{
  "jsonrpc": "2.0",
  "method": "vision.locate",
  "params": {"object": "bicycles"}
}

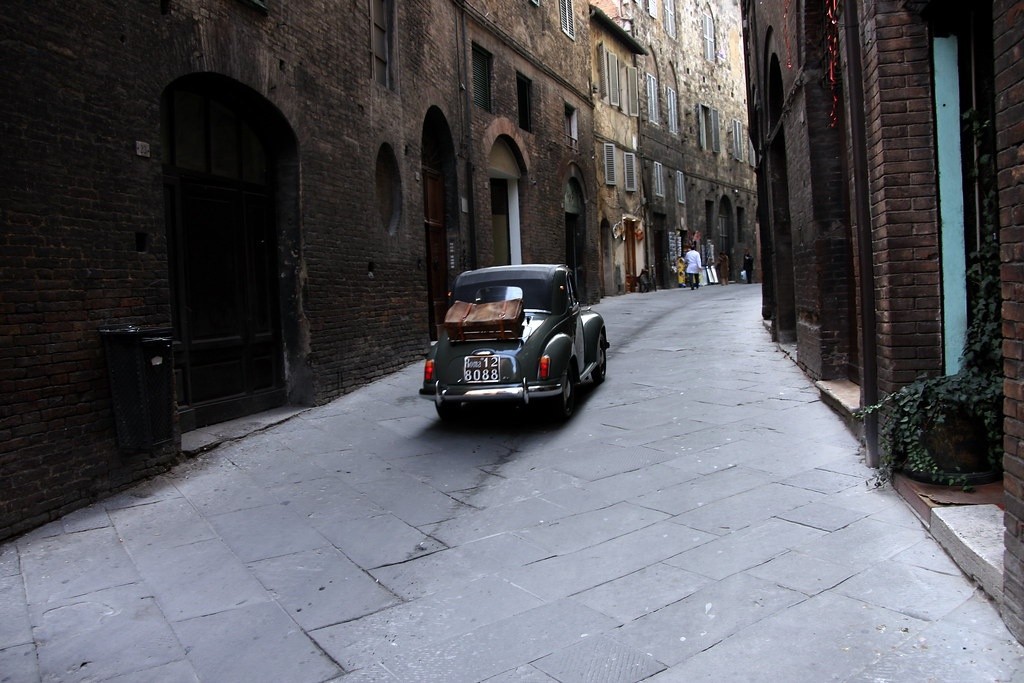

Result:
[636,264,656,292]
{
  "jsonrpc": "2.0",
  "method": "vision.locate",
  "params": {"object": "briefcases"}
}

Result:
[444,298,525,344]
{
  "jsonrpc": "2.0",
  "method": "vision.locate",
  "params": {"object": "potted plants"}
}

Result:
[852,87,1004,492]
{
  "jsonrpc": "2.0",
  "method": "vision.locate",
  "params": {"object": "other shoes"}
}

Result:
[692,285,694,290]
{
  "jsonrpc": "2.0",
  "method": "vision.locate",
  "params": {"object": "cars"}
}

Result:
[417,264,610,423]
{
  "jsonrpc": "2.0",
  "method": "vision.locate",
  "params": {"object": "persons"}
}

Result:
[683,245,702,290]
[716,252,730,285]
[742,248,755,284]
